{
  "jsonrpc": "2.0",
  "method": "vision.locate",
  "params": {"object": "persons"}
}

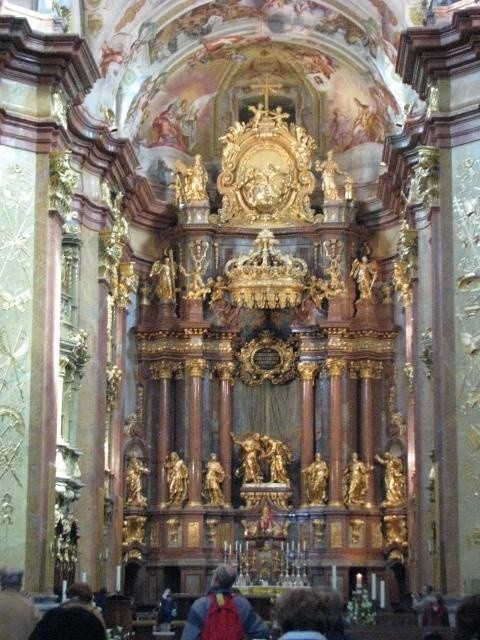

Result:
[316,152,344,201]
[123,436,409,556]
[353,257,374,298]
[181,267,224,299]
[0,564,480,640]
[181,153,209,201]
[155,258,172,300]
[306,269,343,305]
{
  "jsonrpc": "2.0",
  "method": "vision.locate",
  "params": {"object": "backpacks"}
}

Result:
[201,594,244,640]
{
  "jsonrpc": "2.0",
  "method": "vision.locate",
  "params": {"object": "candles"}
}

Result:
[368,573,377,598]
[331,564,338,592]
[354,570,365,593]
[113,565,123,593]
[59,578,66,599]
[379,579,384,609]
[219,537,312,558]
